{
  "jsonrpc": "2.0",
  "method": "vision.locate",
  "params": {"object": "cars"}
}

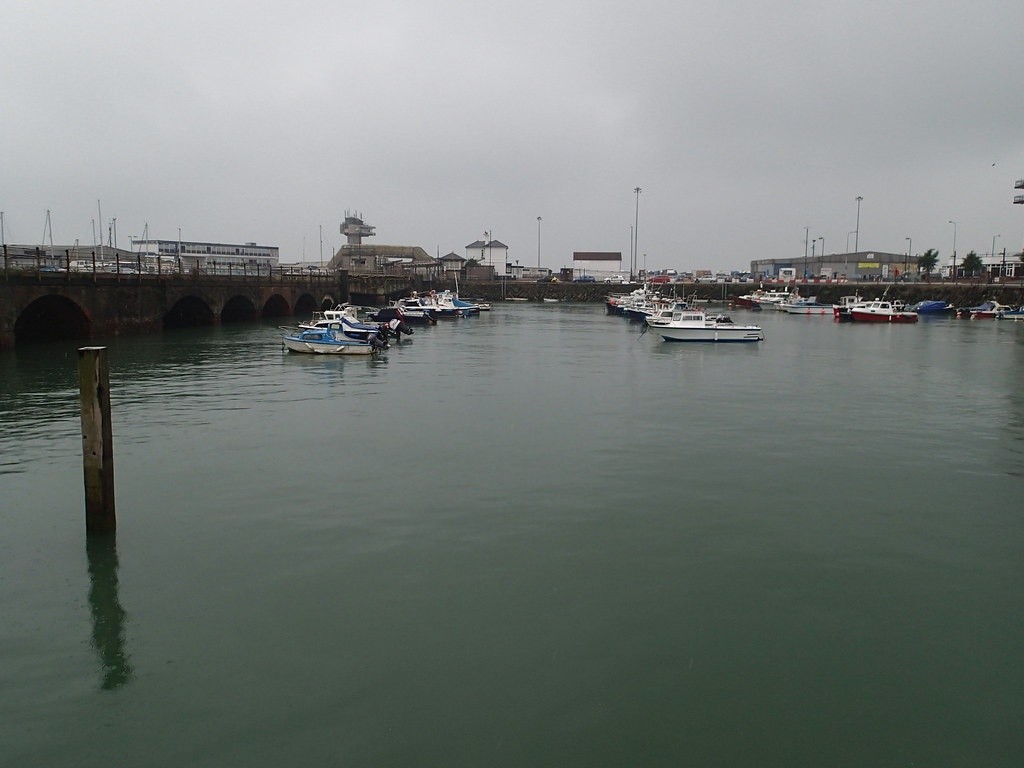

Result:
[693,275,717,284]
[604,275,624,284]
[537,276,557,283]
[572,275,595,283]
[647,275,670,284]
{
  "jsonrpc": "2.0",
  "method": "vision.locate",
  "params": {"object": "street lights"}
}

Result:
[948,220,956,251]
[992,234,1000,256]
[905,237,912,262]
[845,231,858,263]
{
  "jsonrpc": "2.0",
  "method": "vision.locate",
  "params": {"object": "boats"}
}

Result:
[298,310,382,340]
[783,296,833,314]
[650,310,762,341]
[970,305,1013,318]
[774,286,817,311]
[956,301,1000,315]
[622,296,674,320]
[760,291,790,303]
[733,289,766,305]
[616,293,639,315]
[903,301,954,313]
[645,302,716,327]
[284,323,375,354]
[604,292,629,314]
[424,289,480,317]
[833,295,863,316]
[996,306,1024,319]
[365,291,436,324]
[849,280,919,323]
